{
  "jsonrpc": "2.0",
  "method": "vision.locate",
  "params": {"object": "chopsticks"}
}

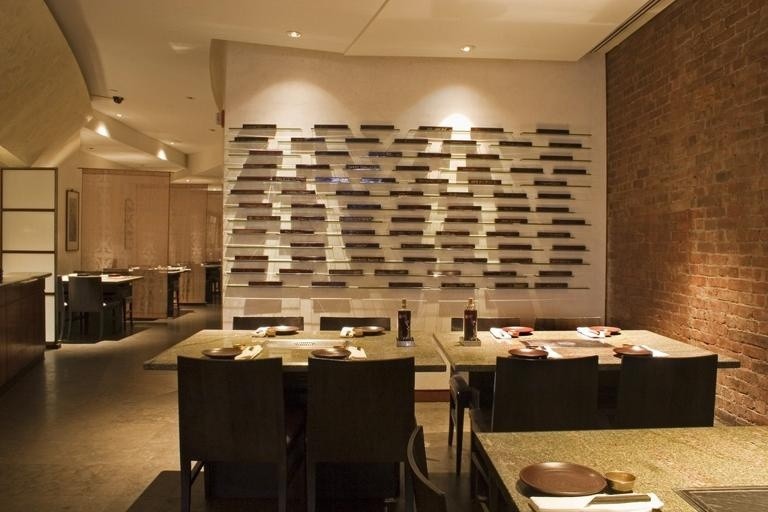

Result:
[591,494,652,503]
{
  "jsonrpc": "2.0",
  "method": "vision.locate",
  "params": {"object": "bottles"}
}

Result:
[397,300,414,341]
[463,298,480,339]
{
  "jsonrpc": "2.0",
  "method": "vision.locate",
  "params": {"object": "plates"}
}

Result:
[509,346,547,359]
[352,325,384,333]
[613,347,650,356]
[590,324,620,335]
[503,325,535,336]
[265,324,297,334]
[310,346,350,358]
[518,460,608,498]
[202,347,242,358]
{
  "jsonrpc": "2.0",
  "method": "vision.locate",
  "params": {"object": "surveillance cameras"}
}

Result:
[113,95,124,104]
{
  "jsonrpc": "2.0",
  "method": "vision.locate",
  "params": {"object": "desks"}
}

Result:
[434,330,741,412]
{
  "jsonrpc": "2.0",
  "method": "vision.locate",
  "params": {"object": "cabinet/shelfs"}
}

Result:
[0,275,48,388]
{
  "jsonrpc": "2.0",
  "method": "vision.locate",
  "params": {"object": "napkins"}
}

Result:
[577,327,604,338]
[530,493,664,511]
[489,327,511,339]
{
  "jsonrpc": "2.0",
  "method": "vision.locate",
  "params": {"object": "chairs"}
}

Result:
[449,319,522,484]
[611,355,717,429]
[55,261,221,344]
[470,355,602,509]
[178,315,449,511]
[534,319,601,331]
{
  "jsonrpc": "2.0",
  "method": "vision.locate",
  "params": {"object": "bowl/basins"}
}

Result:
[604,471,637,492]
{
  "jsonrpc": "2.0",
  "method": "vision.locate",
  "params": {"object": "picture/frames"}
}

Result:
[65,189,79,252]
